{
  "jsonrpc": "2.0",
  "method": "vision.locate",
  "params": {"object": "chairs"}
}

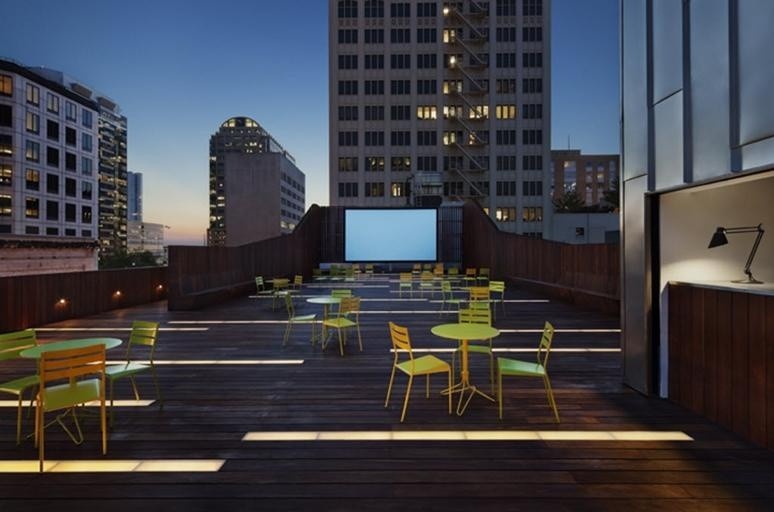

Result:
[0,319,160,474]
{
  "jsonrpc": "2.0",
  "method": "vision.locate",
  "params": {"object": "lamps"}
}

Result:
[706,223,765,285]
